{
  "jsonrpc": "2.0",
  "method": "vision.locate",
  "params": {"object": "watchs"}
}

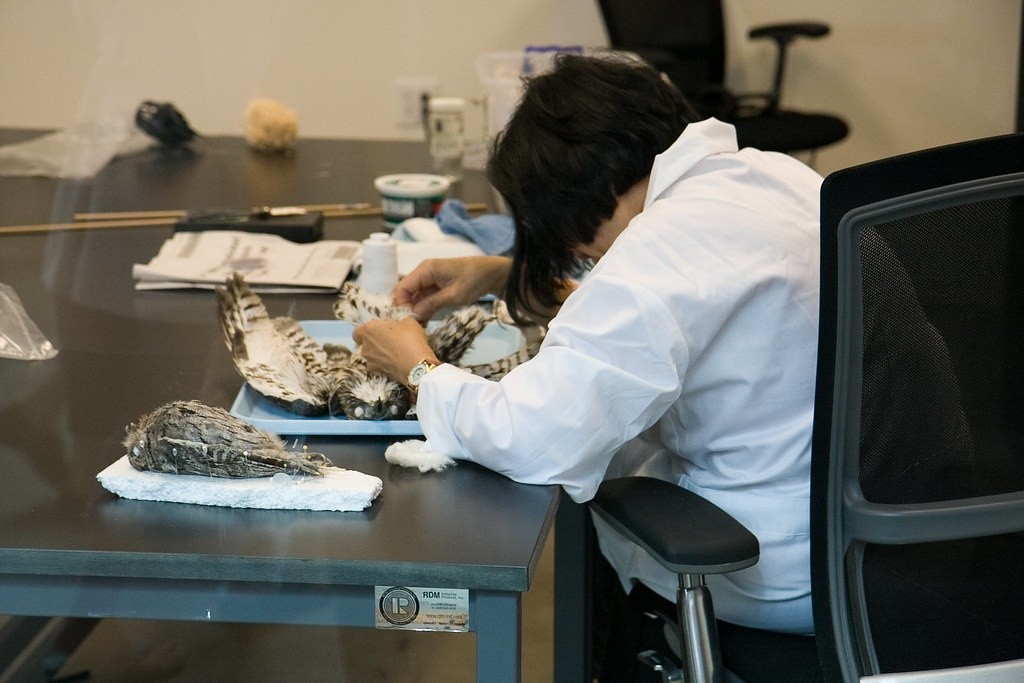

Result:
[407,358,442,402]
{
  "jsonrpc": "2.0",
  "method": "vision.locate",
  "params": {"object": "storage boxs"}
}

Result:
[475,49,646,141]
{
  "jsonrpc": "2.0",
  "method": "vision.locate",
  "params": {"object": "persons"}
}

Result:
[354,50,825,683]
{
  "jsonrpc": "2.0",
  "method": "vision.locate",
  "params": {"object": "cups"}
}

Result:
[354,232,400,322]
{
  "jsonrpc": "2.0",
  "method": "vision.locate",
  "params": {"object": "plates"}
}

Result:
[228,320,521,436]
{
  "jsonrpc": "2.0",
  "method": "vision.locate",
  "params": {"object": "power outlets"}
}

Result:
[390,82,435,126]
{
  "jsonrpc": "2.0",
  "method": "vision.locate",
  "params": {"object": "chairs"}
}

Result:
[592,134,1024,682]
[595,0,849,152]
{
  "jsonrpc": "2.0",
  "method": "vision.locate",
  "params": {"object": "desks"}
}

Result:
[0,126,604,683]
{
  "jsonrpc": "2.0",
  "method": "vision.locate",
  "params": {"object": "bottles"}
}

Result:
[427,97,466,158]
